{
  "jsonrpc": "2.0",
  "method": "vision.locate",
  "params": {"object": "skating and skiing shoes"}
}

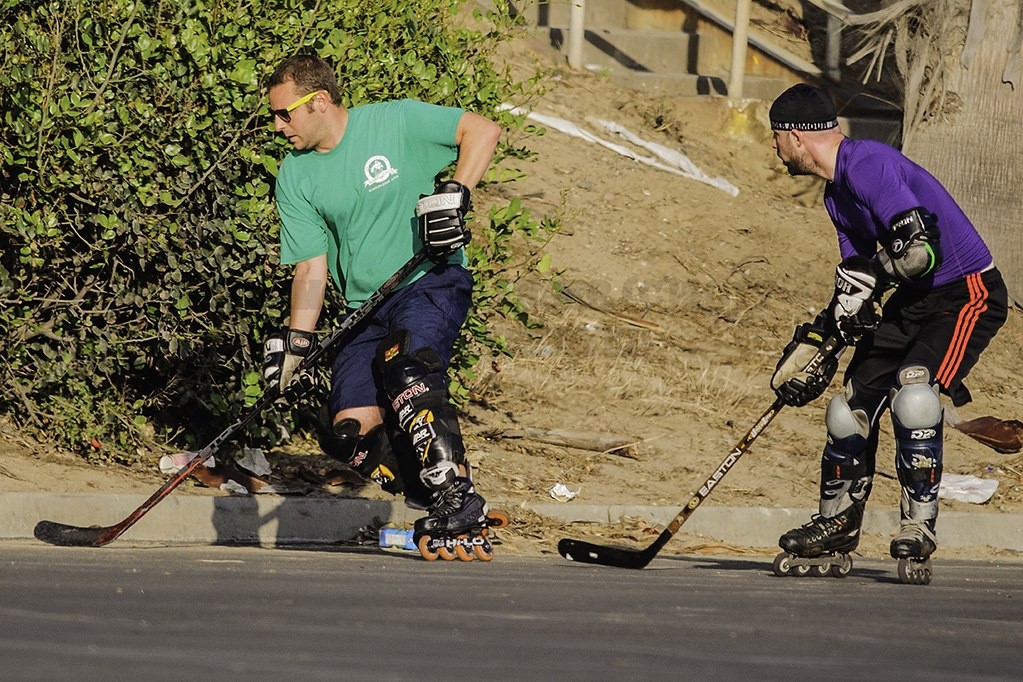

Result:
[476,494,511,531]
[773,513,860,579]
[413,491,490,559]
[890,512,939,585]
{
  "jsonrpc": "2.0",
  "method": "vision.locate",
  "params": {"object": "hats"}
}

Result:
[769,83,838,132]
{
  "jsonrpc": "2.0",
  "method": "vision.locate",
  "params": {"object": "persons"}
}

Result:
[262,53,509,564]
[763,84,1010,585]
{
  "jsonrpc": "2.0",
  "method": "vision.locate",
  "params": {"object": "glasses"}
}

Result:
[266,89,329,123]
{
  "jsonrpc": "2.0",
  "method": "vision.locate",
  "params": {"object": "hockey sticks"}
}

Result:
[554,332,841,571]
[32,246,428,549]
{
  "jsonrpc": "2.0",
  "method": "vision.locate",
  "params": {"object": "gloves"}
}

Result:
[262,326,319,413]
[770,308,848,407]
[414,179,472,266]
[832,255,884,347]
